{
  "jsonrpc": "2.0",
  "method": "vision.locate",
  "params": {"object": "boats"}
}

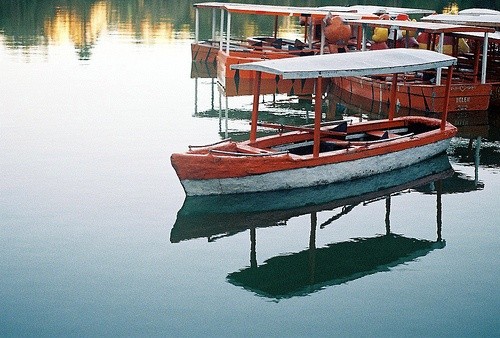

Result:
[217,75,329,141]
[326,82,488,195]
[191,1,500,112]
[191,60,218,119]
[170,48,458,197]
[169,149,456,299]
